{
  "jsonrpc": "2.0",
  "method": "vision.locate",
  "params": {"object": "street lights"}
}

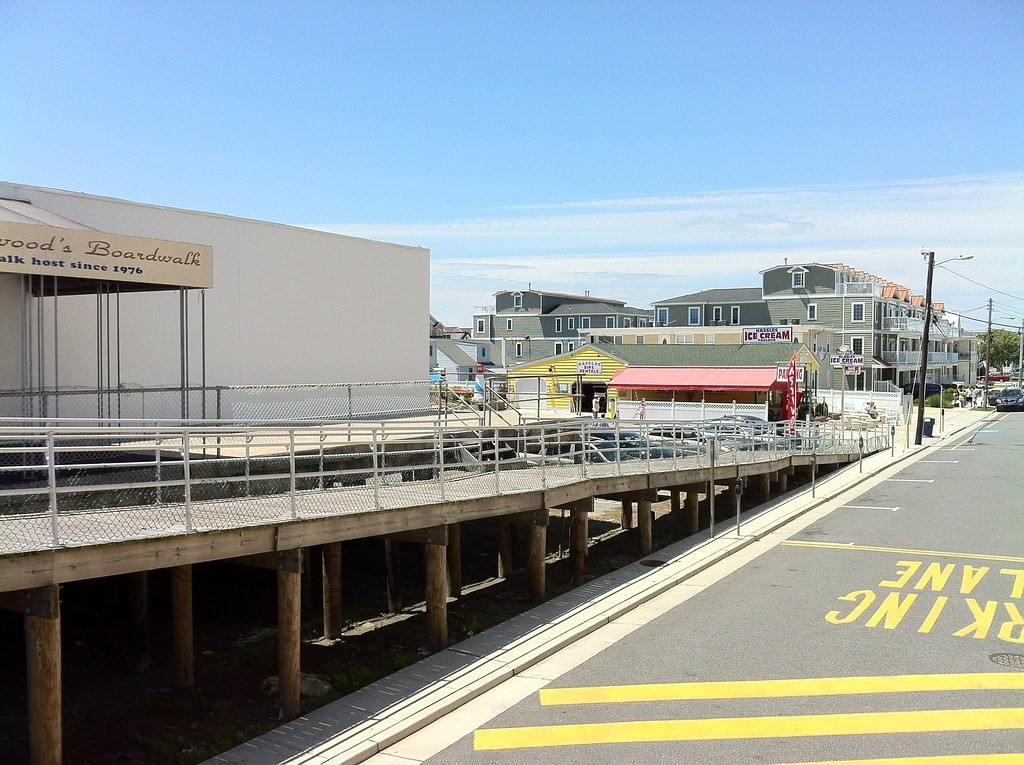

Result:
[983,316,1016,412]
[913,252,974,447]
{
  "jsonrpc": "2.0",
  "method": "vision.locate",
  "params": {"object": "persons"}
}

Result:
[613,410,619,419]
[637,400,645,420]
[592,395,601,419]
[866,401,878,419]
[953,383,986,409]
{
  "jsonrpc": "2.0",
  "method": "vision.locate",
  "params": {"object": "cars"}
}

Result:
[988,386,1024,412]
[977,372,1010,383]
[453,386,472,400]
[900,382,945,400]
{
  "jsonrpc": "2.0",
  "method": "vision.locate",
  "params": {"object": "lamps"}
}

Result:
[662,319,677,327]
[549,365,556,372]
[524,336,530,341]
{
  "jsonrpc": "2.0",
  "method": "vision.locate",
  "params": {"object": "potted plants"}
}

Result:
[462,397,473,409]
[466,387,474,398]
[447,392,455,402]
[450,385,462,391]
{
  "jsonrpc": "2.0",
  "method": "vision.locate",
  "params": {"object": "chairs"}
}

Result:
[827,413,887,432]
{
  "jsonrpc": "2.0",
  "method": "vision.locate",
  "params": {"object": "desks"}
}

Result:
[869,409,886,418]
[855,414,869,422]
[844,416,860,426]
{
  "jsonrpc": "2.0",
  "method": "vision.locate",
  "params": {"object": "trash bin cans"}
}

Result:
[923,417,935,437]
